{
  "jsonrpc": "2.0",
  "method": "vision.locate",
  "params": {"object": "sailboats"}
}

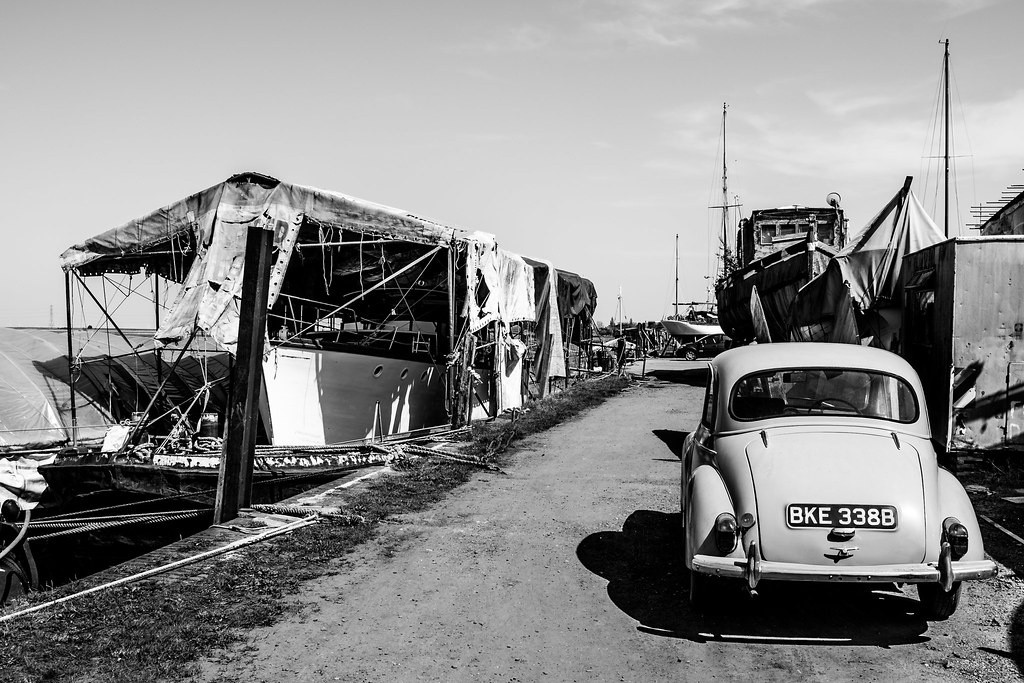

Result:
[662,102,729,341]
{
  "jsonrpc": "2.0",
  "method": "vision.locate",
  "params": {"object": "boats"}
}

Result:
[710,205,853,337]
[39,192,631,504]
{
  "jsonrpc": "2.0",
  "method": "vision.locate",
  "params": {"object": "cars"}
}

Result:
[681,343,997,619]
[675,334,736,360]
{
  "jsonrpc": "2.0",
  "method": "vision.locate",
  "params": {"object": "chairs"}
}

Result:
[732,397,784,419]
